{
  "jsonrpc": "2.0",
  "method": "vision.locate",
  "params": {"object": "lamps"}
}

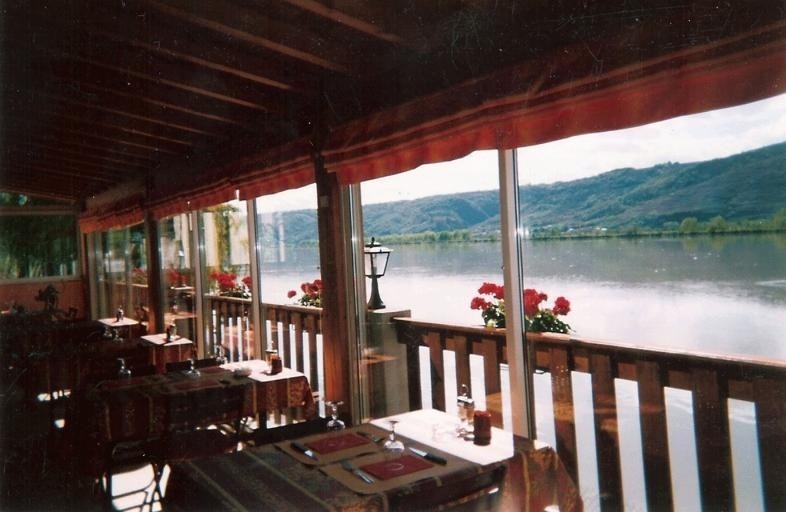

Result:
[363,237,394,310]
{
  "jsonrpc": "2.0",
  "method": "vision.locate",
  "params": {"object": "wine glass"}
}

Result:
[323,398,345,446]
[103,324,225,388]
[383,417,405,472]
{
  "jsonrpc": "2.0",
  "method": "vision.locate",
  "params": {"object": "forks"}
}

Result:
[356,429,385,443]
[342,459,375,485]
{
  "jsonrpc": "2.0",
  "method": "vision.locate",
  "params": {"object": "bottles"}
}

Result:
[453,385,492,446]
[115,305,126,322]
[263,341,282,375]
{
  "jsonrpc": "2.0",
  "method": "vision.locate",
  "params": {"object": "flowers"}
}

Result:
[287,279,323,308]
[471,282,577,336]
[208,269,253,299]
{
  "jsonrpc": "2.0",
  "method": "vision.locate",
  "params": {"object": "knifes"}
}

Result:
[408,444,448,466]
[289,439,319,462]
